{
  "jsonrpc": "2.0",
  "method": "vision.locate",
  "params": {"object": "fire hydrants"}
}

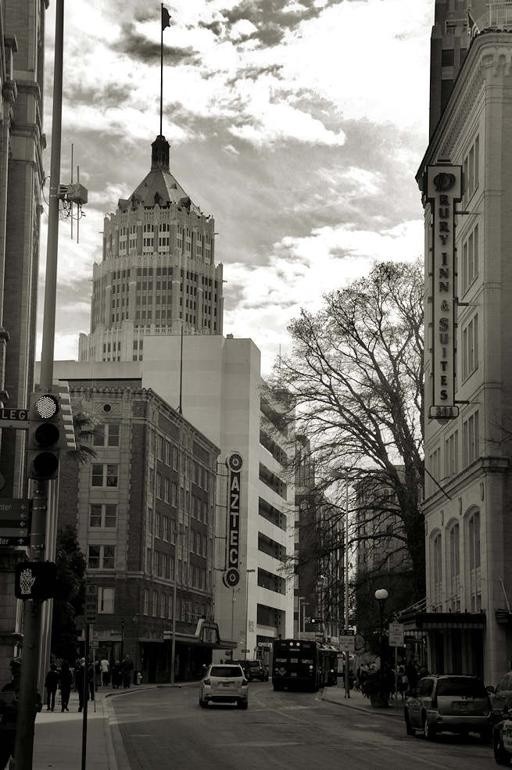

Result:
[137,672,143,684]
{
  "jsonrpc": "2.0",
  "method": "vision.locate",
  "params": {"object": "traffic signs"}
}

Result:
[0,497,29,545]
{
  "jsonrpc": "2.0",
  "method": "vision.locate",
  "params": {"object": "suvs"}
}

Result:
[199,658,269,709]
[402,672,494,741]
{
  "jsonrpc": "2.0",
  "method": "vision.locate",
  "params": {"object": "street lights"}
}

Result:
[373,585,395,710]
[328,469,351,692]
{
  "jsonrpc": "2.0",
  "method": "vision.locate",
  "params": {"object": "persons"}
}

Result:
[347,660,430,701]
[45,655,134,712]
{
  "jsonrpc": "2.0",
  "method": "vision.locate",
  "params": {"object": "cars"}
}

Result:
[487,670,512,767]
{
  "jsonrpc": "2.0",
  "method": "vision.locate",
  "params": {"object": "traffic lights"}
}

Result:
[13,559,61,601]
[25,391,63,482]
[310,616,321,624]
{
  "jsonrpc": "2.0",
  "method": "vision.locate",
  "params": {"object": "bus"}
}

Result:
[271,638,341,693]
